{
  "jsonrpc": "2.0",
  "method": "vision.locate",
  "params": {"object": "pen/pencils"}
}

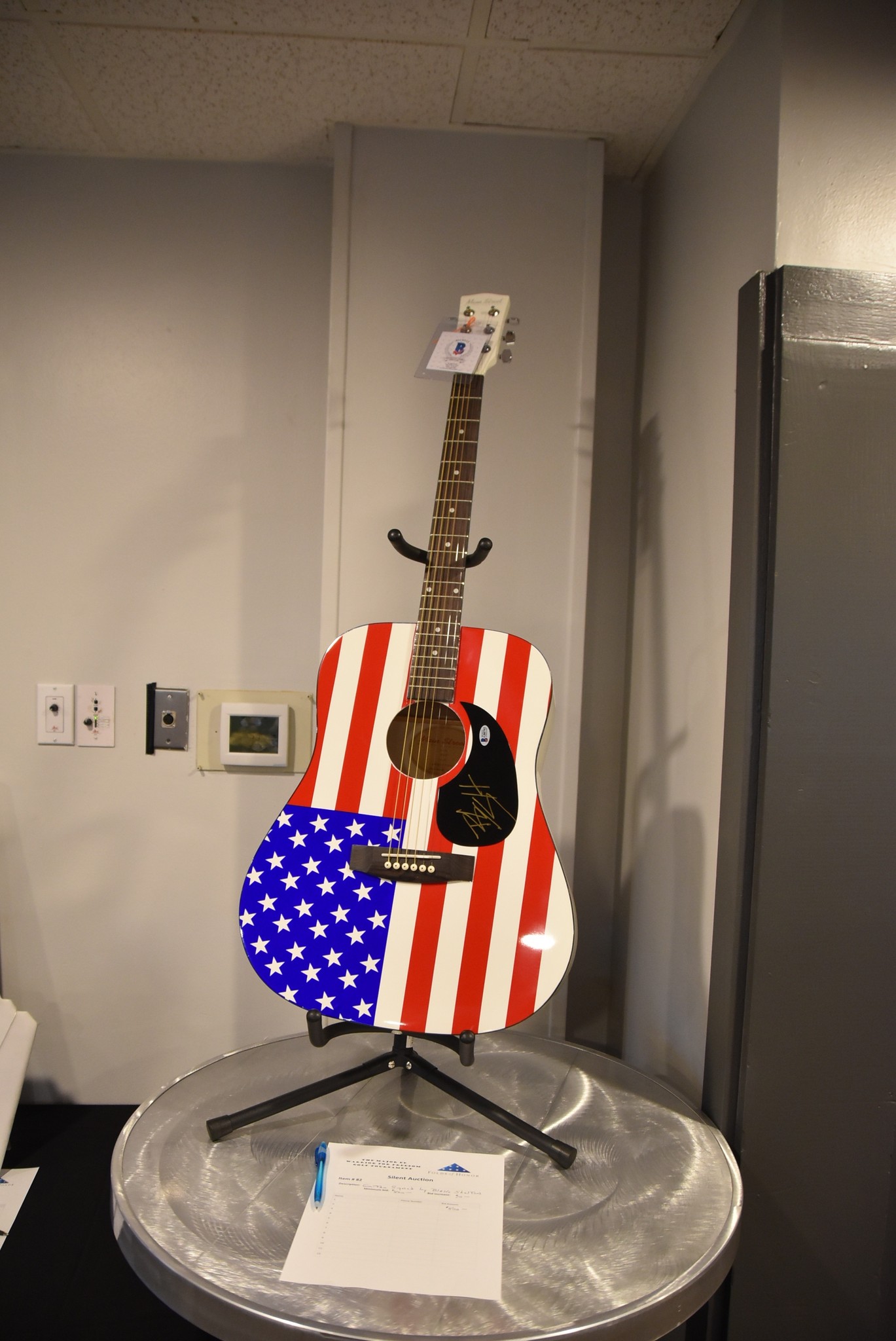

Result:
[314,1141,327,1210]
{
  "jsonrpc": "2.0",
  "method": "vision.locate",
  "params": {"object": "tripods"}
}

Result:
[205,526,580,1167]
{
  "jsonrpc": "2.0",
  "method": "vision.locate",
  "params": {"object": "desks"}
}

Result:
[113,1032,745,1341]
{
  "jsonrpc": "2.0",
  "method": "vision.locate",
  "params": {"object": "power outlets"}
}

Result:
[36,685,189,753]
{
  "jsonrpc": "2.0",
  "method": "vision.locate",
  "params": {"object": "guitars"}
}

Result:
[235,290,577,1046]
